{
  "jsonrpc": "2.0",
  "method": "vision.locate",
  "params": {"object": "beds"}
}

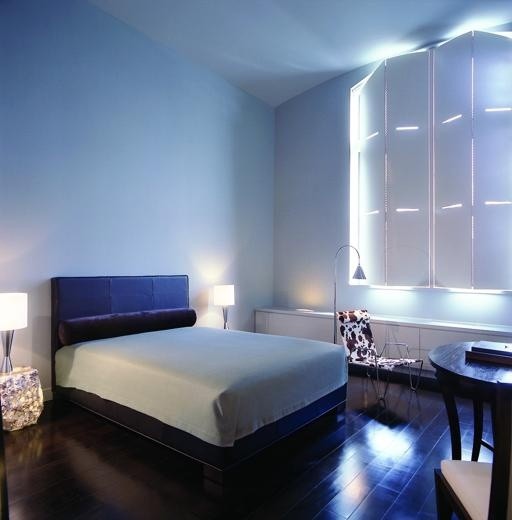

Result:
[51,275,347,484]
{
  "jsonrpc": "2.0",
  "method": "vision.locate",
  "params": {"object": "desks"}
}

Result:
[428,342,512,461]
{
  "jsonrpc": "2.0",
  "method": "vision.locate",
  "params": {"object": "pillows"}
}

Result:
[144,308,197,332]
[58,312,144,345]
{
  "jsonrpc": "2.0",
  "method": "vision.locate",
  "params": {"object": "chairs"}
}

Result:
[435,383,512,520]
[336,309,423,400]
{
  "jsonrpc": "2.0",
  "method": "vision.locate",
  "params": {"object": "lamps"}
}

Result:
[214,285,234,330]
[0,293,27,373]
[334,244,366,344]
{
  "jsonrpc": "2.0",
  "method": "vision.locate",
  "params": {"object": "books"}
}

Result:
[464,342,512,366]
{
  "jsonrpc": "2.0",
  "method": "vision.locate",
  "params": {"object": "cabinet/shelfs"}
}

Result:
[253,307,512,382]
